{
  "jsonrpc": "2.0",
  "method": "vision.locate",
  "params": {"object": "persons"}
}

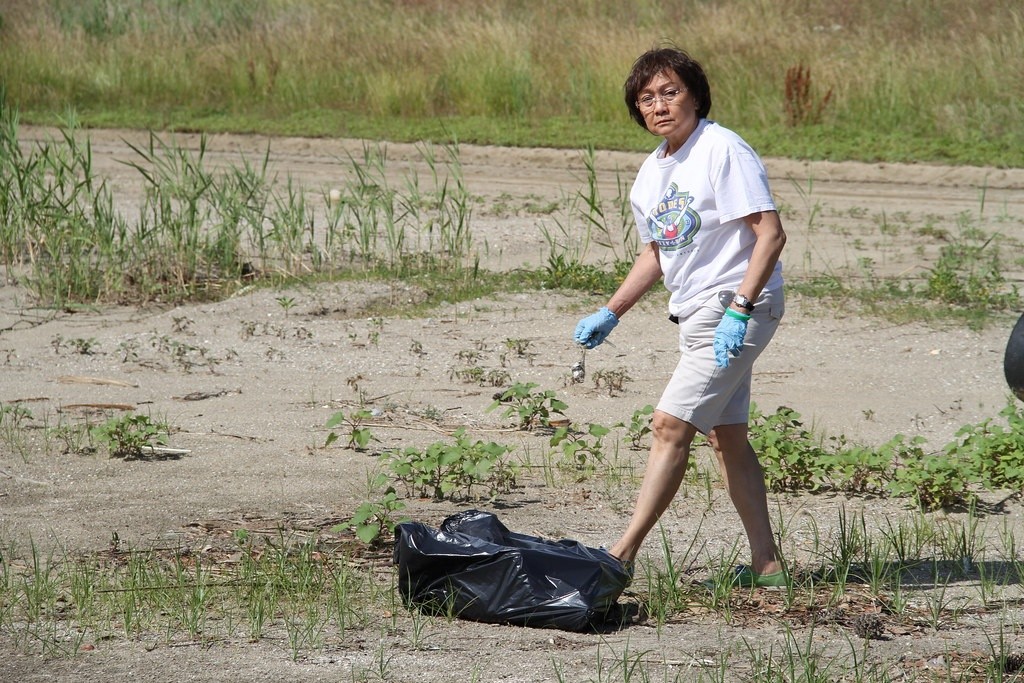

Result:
[575,48,789,589]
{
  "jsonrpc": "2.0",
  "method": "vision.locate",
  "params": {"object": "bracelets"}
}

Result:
[726,307,751,321]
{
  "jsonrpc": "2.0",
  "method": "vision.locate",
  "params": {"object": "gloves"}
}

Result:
[712,306,751,368]
[573,306,620,349]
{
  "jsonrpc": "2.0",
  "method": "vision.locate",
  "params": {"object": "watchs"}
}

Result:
[733,295,755,312]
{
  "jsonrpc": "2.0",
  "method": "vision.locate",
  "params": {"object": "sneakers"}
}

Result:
[621,561,635,587]
[704,565,792,591]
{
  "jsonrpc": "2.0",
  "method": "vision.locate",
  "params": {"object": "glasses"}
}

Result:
[634,87,689,109]
